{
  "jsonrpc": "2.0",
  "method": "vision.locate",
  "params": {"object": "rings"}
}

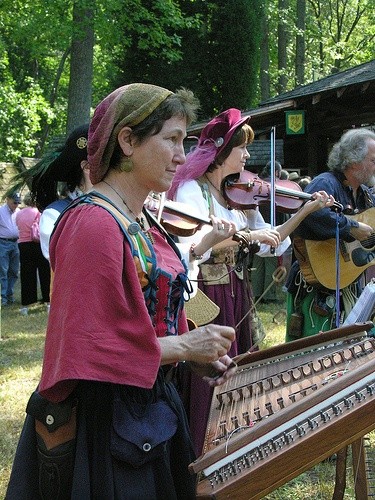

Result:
[218,226,223,230]
[225,227,230,230]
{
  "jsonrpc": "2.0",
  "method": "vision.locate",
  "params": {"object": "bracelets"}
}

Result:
[190,243,203,260]
[244,228,253,243]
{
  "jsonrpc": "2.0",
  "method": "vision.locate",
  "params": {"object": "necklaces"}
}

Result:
[101,179,155,245]
[204,172,233,210]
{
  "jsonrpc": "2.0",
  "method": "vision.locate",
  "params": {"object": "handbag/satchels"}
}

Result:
[25,390,80,500]
[30,212,41,242]
[109,382,179,468]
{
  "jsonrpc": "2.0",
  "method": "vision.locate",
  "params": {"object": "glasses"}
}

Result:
[14,202,21,204]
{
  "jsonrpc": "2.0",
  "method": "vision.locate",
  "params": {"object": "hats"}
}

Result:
[87,83,174,184]
[8,192,21,201]
[198,108,251,160]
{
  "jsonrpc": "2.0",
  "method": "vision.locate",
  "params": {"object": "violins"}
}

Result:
[225,168,354,218]
[143,195,261,256]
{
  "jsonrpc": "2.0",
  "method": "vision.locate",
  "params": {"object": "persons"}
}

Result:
[31,124,236,270]
[166,109,334,459]
[283,128,375,459]
[251,160,286,304]
[277,169,311,288]
[5,83,238,500]
[0,192,21,306]
[16,190,50,315]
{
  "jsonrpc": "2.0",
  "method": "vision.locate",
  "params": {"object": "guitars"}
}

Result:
[291,207,375,290]
